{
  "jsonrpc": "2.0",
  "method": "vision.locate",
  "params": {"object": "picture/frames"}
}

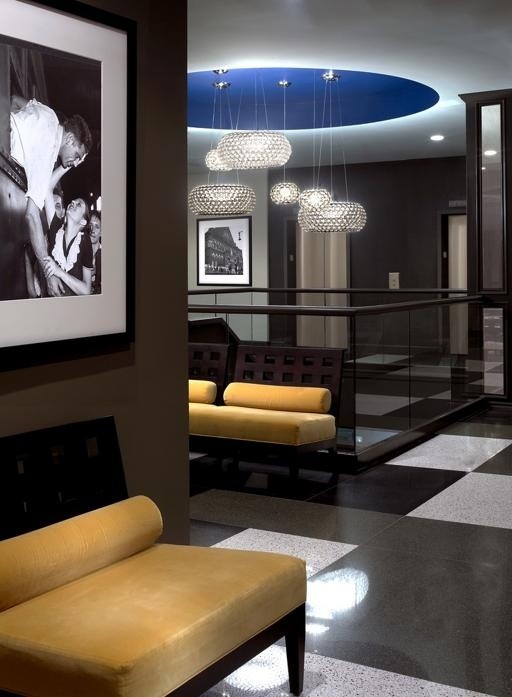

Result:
[0,0,140,373]
[195,214,253,288]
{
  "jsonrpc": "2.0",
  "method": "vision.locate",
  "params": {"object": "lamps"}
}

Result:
[188,65,368,233]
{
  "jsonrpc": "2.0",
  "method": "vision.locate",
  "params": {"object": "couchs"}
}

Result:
[1,414,307,695]
[188,340,345,488]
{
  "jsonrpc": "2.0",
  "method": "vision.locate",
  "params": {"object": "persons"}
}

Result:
[38,149,94,296]
[86,208,102,294]
[52,184,66,222]
[8,94,94,299]
